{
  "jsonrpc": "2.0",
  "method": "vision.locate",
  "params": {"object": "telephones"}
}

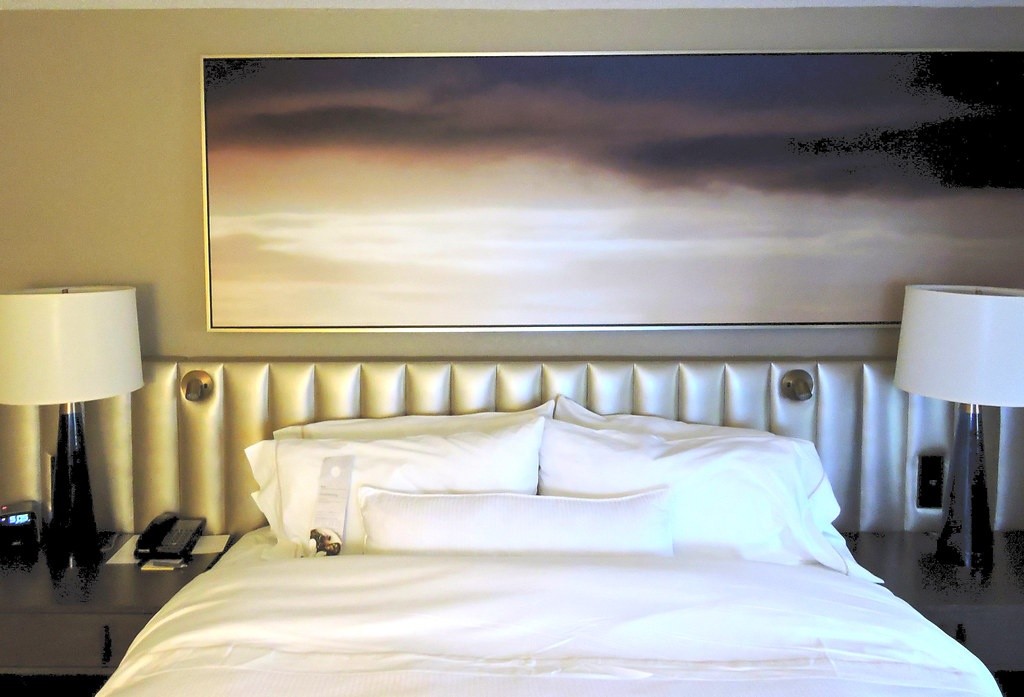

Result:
[133,510,207,560]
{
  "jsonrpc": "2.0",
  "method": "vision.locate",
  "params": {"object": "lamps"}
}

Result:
[891,283,1024,568]
[0,282,146,565]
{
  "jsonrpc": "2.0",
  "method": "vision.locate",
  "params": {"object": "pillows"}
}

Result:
[244,393,888,585]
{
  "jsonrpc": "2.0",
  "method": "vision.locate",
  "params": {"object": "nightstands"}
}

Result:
[0,532,237,697]
[840,530,1024,697]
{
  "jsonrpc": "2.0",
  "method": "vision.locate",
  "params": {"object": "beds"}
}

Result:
[94,522,1006,697]
